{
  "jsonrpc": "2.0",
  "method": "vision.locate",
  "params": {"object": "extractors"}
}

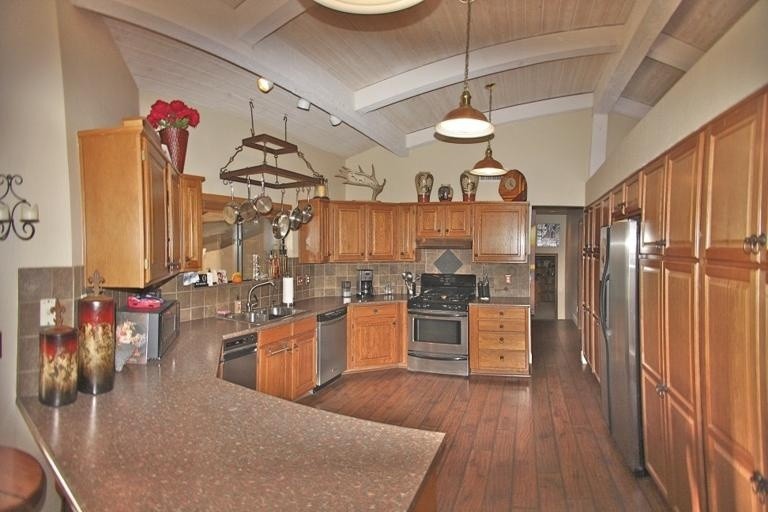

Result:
[416,237,472,249]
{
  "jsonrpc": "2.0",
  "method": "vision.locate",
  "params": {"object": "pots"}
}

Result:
[222,173,314,241]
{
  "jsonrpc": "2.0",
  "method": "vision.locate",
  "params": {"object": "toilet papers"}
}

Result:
[283,277,293,304]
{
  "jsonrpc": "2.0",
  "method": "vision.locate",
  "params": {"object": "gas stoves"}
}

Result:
[407,290,473,310]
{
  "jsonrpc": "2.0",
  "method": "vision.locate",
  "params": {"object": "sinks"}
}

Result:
[213,312,283,325]
[252,305,311,317]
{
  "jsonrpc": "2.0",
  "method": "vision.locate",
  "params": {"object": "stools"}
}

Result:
[0,445,46,512]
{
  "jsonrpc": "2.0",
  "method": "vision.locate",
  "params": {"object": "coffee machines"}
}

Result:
[355,269,374,296]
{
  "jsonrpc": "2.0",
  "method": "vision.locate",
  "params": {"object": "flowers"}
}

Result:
[147,100,200,129]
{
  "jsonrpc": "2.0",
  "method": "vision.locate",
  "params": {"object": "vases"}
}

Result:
[158,127,189,174]
[460,170,479,202]
[438,184,453,202]
[415,172,434,203]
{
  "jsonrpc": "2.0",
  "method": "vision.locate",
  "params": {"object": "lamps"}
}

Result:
[468,82,509,178]
[0,174,39,241]
[329,114,342,126]
[296,99,311,111]
[434,1,494,141]
[257,77,273,93]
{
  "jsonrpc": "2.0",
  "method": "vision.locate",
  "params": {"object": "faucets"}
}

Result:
[246,281,276,310]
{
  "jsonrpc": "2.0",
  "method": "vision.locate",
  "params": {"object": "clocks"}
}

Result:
[499,170,527,202]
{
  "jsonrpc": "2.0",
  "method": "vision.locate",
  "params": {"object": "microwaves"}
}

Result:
[115,299,181,360]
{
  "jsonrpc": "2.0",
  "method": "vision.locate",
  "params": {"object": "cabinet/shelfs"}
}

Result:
[254,316,317,401]
[472,201,530,262]
[416,202,472,240]
[346,301,407,374]
[78,116,167,288]
[167,160,181,277]
[330,201,365,264]
[469,303,531,378]
[365,202,396,263]
[298,200,331,264]
[395,203,421,263]
[181,173,207,273]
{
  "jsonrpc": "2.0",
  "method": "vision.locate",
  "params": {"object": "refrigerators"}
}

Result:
[599,218,645,477]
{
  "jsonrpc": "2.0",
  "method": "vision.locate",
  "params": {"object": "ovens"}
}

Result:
[407,308,469,377]
